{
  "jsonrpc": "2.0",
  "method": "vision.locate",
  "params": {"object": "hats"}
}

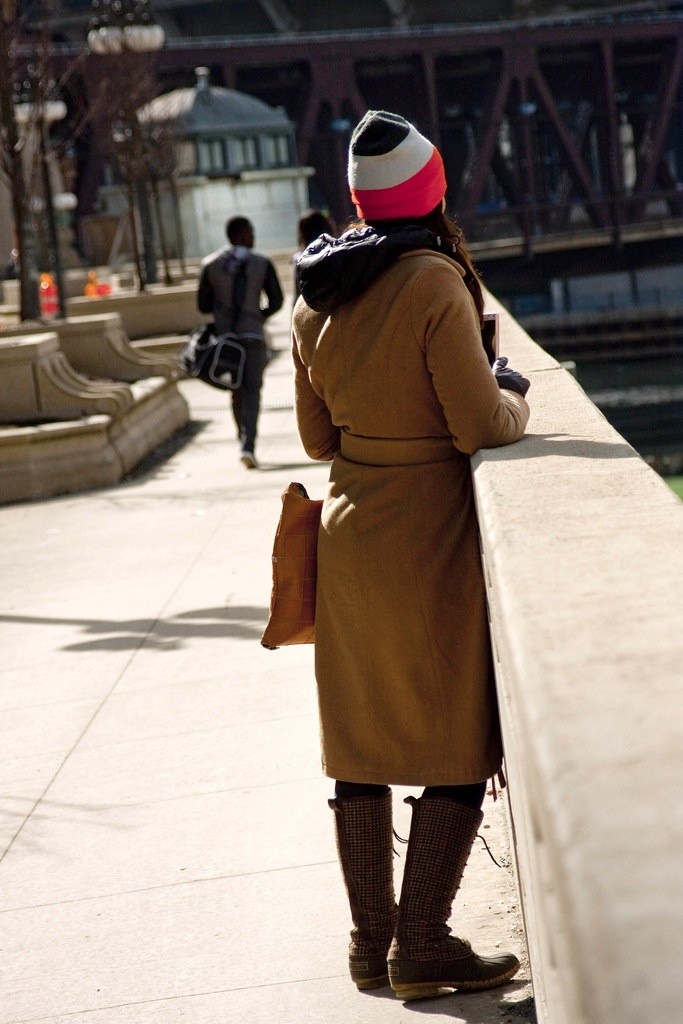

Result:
[348,110,447,220]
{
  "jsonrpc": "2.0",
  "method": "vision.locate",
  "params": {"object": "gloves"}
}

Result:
[491,357,531,399]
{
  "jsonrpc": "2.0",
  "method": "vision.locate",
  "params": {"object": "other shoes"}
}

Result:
[239,445,258,467]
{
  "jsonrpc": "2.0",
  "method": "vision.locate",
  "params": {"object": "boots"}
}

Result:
[327,790,400,990]
[385,795,519,998]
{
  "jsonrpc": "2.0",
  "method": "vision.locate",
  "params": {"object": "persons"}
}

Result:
[194,215,286,469]
[293,213,334,262]
[293,105,534,1007]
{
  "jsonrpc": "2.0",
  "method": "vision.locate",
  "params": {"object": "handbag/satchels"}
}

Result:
[260,482,324,650]
[178,334,245,390]
